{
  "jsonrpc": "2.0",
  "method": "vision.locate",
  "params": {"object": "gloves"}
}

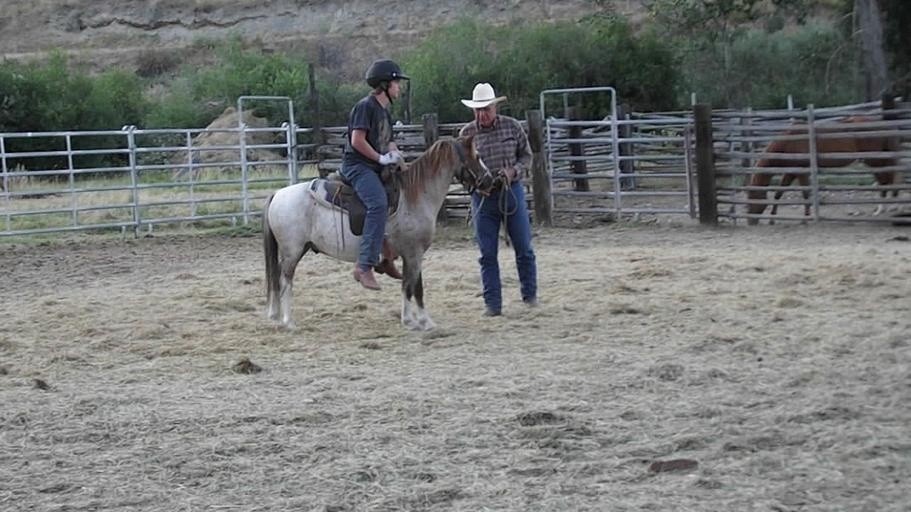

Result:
[378,150,405,167]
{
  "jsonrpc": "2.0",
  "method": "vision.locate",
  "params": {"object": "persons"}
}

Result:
[455,81,543,319]
[337,59,413,292]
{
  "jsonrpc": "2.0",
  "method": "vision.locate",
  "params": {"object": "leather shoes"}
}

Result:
[525,299,538,308]
[377,260,405,282]
[482,309,501,317]
[351,262,383,294]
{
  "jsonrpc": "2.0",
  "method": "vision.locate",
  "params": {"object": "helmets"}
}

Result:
[363,59,412,83]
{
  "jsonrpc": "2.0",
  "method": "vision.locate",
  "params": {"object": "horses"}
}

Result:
[263,134,495,331]
[747,116,899,224]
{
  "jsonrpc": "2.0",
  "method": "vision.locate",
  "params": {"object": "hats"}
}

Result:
[460,82,507,111]
